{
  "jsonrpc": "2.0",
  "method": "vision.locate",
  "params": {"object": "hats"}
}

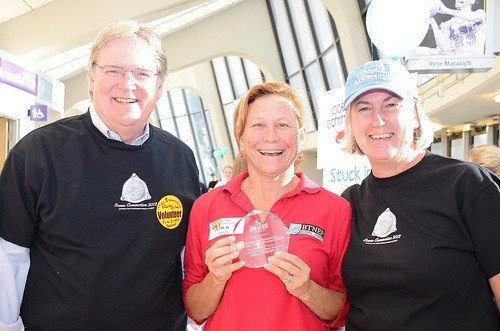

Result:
[343,59,420,110]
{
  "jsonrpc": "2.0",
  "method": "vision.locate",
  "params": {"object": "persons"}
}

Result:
[466,145,500,178]
[176,83,352,330]
[429,0,487,59]
[1,22,218,330]
[331,60,500,330]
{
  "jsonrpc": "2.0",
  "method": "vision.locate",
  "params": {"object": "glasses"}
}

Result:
[93,62,160,82]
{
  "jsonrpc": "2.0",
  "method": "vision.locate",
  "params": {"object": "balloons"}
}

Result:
[364,0,430,62]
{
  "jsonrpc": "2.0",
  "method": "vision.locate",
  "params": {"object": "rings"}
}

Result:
[283,276,293,282]
